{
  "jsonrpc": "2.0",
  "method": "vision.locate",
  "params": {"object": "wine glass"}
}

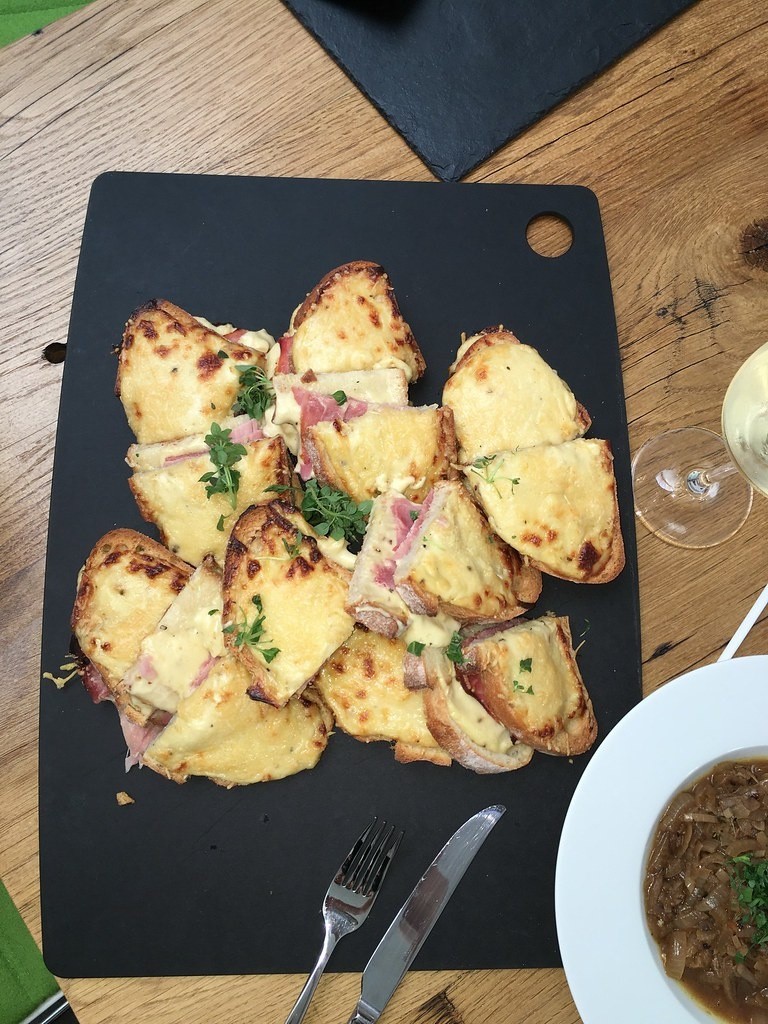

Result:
[627,337,768,550]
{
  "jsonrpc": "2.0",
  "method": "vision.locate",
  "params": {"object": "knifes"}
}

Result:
[339,803,508,1024]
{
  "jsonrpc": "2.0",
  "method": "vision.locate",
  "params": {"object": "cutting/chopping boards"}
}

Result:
[35,174,641,978]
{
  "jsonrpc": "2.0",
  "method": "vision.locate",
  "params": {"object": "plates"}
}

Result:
[554,654,768,1023]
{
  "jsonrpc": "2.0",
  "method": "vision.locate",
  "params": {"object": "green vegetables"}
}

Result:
[198,365,531,690]
[727,854,768,963]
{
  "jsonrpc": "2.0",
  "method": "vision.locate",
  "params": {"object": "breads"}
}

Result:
[318,620,452,768]
[423,634,532,771]
[463,441,625,583]
[305,408,456,510]
[457,617,598,756]
[396,480,543,619]
[66,528,196,720]
[116,298,268,443]
[291,262,426,382]
[140,657,331,788]
[128,429,305,565]
[443,331,592,454]
[220,500,360,710]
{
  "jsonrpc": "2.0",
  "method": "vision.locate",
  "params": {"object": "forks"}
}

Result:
[278,816,406,1024]
